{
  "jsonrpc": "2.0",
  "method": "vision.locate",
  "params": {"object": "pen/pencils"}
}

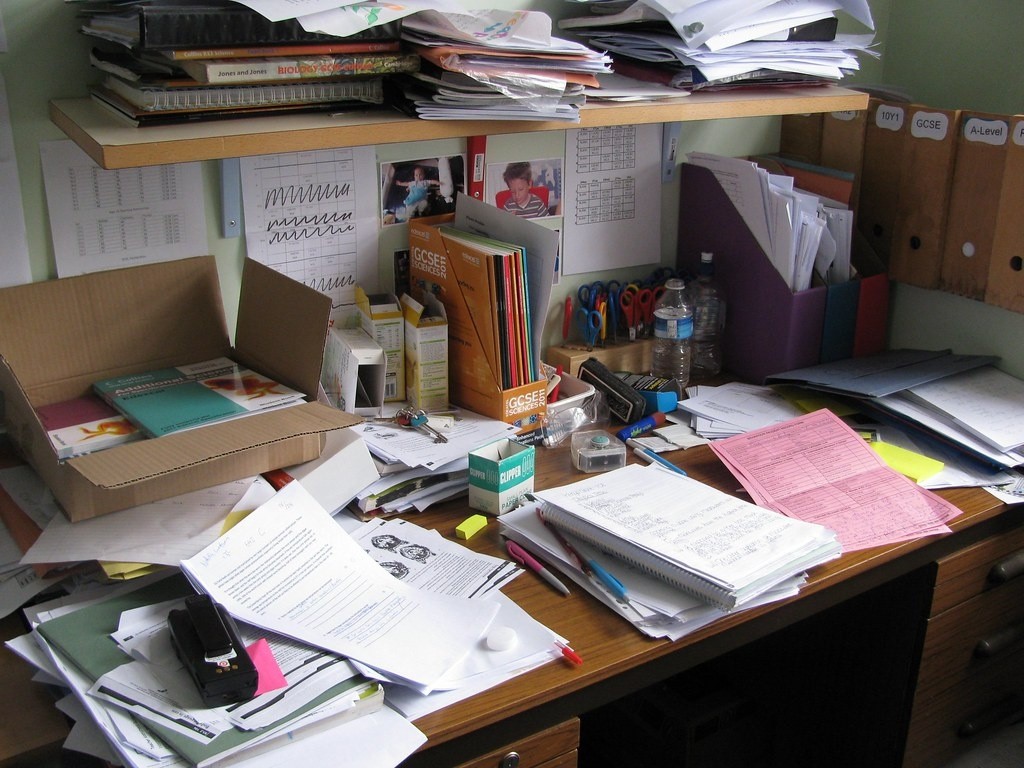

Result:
[554,637,582,665]
[535,506,631,603]
[626,437,687,476]
[505,540,573,598]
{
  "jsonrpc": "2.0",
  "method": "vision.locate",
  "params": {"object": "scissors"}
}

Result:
[560,267,695,350]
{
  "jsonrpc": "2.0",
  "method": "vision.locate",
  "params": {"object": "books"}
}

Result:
[23,568,384,767]
[35,356,310,461]
[350,454,471,514]
[83,0,421,129]
[540,462,843,612]
[434,223,534,391]
[863,391,1024,472]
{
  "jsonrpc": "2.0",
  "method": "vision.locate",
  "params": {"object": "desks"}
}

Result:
[0,445,1023,768]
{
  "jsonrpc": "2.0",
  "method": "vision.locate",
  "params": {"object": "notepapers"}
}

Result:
[867,441,945,484]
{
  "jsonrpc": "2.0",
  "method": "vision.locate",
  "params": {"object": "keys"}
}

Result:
[398,413,448,444]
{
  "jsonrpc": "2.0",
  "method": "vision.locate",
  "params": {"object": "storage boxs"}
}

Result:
[468,439,535,514]
[326,326,386,419]
[408,212,549,451]
[401,291,449,414]
[679,154,890,384]
[0,254,365,521]
[352,284,407,403]
[616,371,681,415]
[545,363,594,416]
[779,103,1023,314]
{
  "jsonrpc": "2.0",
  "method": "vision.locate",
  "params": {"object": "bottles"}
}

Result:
[650,278,694,389]
[688,251,726,379]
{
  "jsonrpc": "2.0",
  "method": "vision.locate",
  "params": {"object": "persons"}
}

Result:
[503,162,551,218]
[395,166,445,219]
